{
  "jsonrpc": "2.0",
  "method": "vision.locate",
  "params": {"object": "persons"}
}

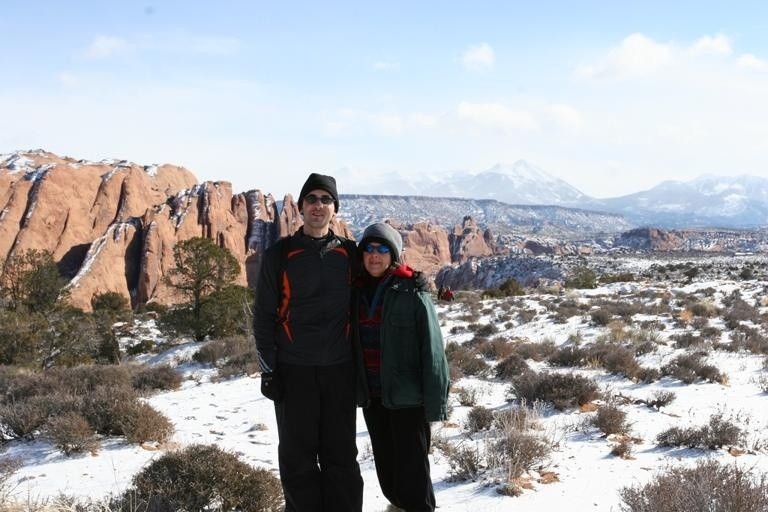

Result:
[251,171,434,510]
[438,283,445,300]
[348,220,451,510]
[441,285,454,301]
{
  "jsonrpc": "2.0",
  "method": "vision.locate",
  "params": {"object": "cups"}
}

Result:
[305,194,333,204]
[363,244,391,254]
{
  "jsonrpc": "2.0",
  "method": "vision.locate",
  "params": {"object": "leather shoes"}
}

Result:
[413,271,431,291]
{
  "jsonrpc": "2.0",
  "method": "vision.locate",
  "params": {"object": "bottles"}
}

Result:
[357,223,403,262]
[297,173,339,215]
